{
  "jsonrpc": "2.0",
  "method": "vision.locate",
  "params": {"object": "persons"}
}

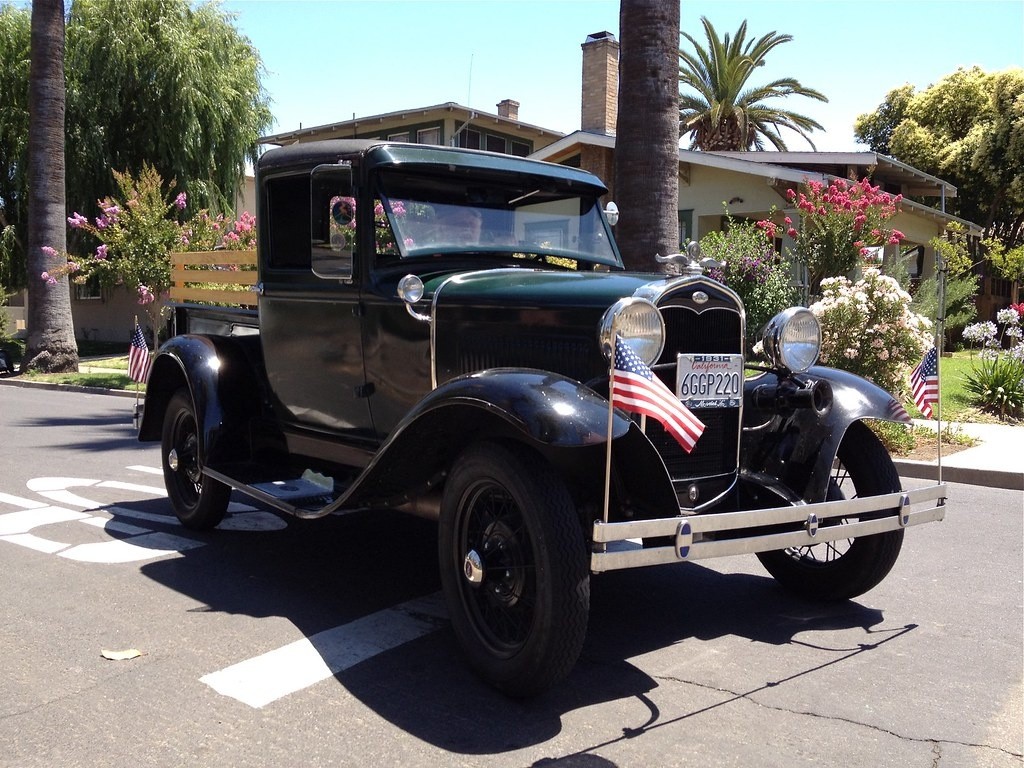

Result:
[436,205,519,247]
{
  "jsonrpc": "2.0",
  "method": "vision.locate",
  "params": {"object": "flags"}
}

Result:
[127,322,153,383]
[608,333,707,455]
[909,344,941,418]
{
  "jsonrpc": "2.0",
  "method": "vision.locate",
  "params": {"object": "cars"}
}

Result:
[132,137,950,709]
